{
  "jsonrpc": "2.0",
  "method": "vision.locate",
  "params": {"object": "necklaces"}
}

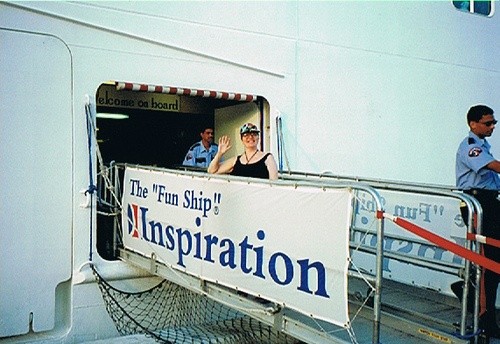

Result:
[244,149,258,165]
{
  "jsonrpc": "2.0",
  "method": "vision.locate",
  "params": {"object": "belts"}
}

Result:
[464,189,498,197]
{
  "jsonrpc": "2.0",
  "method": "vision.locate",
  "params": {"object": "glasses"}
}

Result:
[477,120,498,126]
[242,133,258,137]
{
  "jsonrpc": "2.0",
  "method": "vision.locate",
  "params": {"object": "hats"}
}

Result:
[239,123,260,133]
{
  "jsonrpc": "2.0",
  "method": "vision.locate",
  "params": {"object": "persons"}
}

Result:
[181,125,225,172]
[455,104,500,341]
[206,122,279,304]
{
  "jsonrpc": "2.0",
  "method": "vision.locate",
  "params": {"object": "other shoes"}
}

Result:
[482,317,500,338]
[450,281,474,313]
